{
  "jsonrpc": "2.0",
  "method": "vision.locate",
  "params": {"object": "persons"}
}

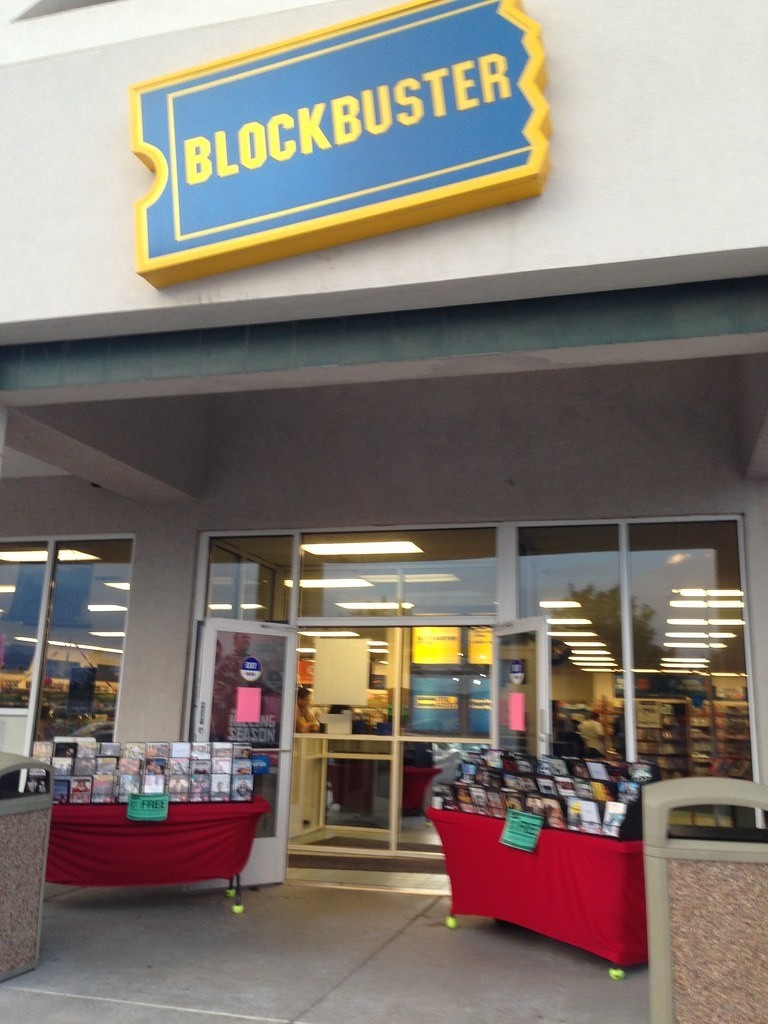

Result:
[296,687,320,733]
[552,720,588,759]
[63,747,75,757]
[581,712,608,757]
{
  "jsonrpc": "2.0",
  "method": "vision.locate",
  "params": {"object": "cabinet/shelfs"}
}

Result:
[692,700,713,763]
[601,694,624,759]
[635,696,692,782]
[712,699,751,779]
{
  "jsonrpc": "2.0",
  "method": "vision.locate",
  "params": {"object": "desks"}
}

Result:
[42,794,272,915]
[425,805,645,981]
[326,763,442,818]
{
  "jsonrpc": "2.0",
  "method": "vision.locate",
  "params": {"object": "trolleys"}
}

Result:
[425,804,649,984]
[47,791,272,914]
